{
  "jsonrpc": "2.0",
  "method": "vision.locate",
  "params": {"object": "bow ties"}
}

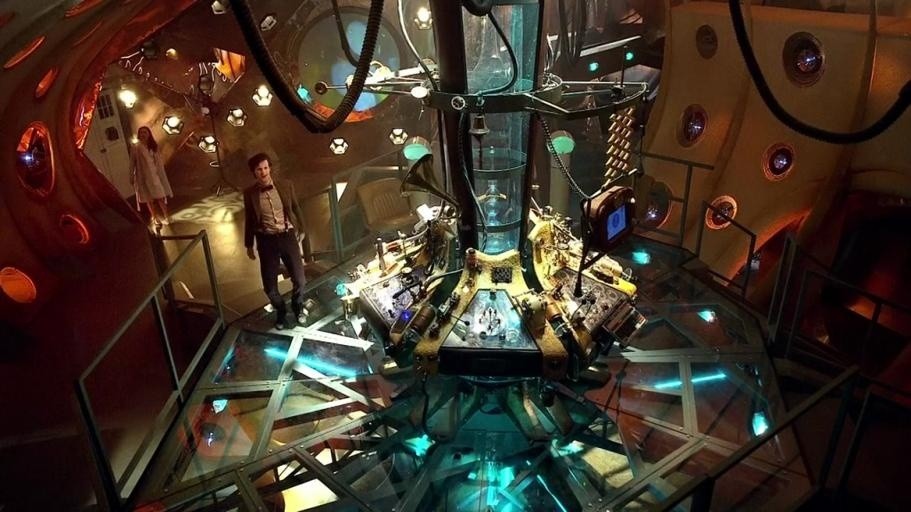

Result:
[260,185,273,193]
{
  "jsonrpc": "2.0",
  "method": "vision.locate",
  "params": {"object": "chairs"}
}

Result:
[356,177,420,270]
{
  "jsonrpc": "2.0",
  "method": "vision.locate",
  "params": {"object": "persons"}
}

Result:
[243,152,309,331]
[128,126,174,226]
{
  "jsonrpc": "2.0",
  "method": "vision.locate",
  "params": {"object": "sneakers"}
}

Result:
[296,313,306,324]
[166,216,174,225]
[153,218,162,225]
[276,317,284,330]
